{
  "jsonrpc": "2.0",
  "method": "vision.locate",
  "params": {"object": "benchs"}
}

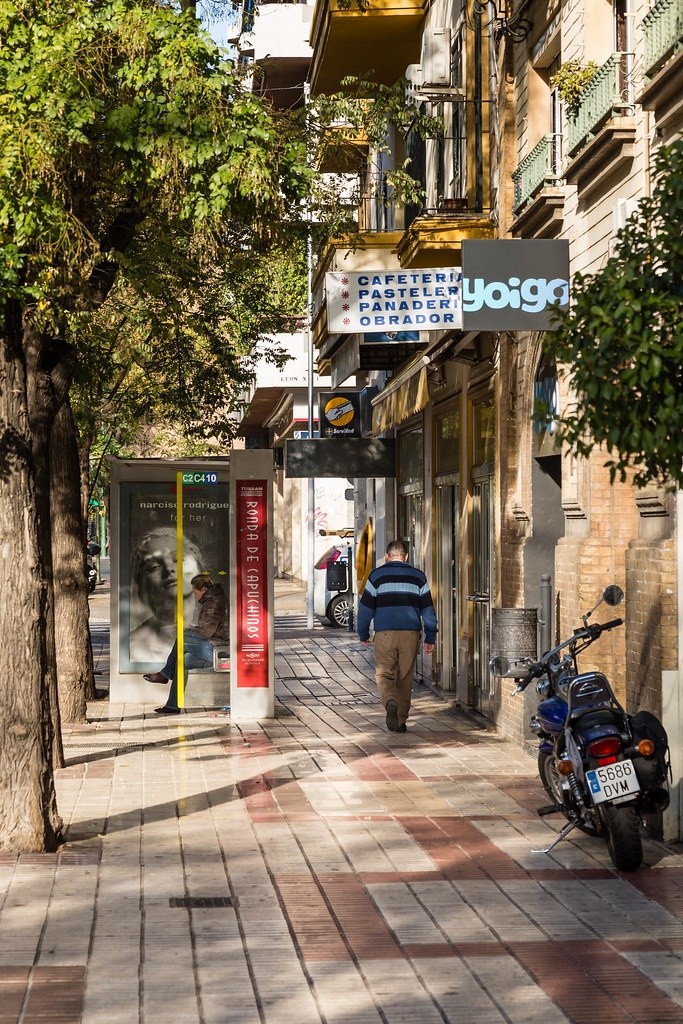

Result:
[212,646,230,673]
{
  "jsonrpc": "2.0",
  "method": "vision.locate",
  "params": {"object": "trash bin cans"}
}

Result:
[491,607,539,679]
[327,561,346,591]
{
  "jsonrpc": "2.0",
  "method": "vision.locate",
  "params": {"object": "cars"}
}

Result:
[306,542,356,628]
[87,543,101,592]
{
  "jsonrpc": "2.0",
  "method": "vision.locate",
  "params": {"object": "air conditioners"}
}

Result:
[420,27,454,87]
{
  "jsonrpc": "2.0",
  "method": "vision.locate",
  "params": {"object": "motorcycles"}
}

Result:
[488,584,674,873]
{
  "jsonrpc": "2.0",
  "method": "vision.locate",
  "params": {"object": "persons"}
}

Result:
[143,574,232,713]
[356,540,439,732]
[129,527,207,663]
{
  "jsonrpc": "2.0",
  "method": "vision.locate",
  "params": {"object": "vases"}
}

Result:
[447,198,466,209]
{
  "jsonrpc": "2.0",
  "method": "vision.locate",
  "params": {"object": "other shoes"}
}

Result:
[143,674,169,684]
[386,701,399,731]
[399,723,407,733]
[154,707,181,713]
